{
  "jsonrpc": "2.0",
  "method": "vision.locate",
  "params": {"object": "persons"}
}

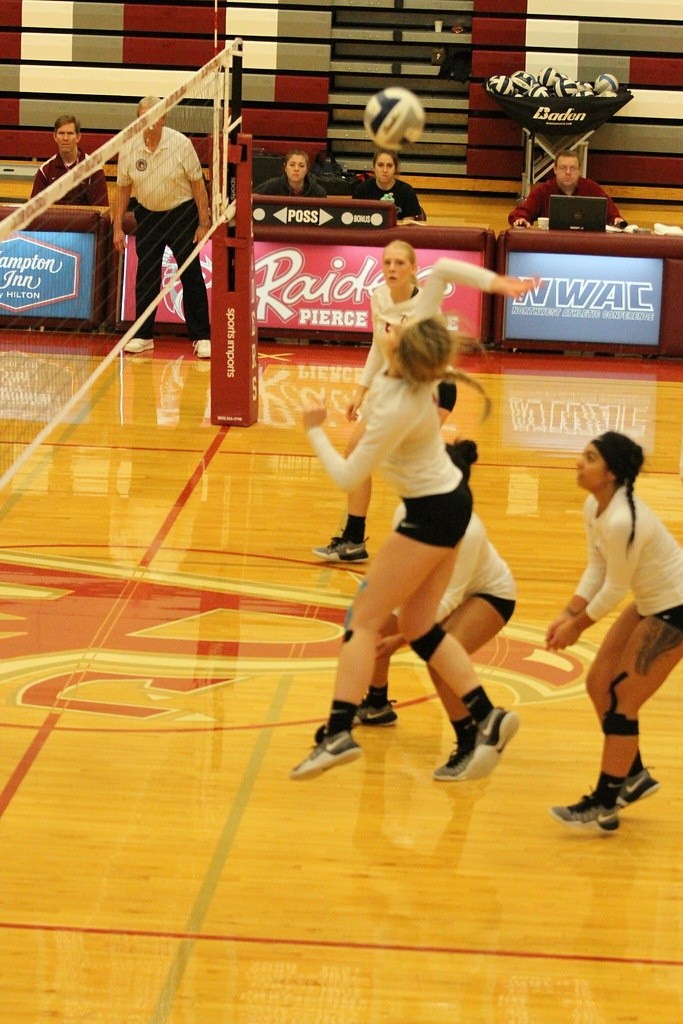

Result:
[311,241,534,562]
[29,114,109,206]
[289,318,521,781]
[545,431,683,832]
[508,150,625,228]
[113,96,211,357]
[352,148,422,221]
[352,439,516,780]
[252,150,327,198]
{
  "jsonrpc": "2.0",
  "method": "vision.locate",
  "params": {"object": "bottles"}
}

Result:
[380,193,395,203]
[342,165,347,179]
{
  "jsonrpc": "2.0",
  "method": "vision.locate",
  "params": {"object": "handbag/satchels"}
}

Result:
[310,149,343,179]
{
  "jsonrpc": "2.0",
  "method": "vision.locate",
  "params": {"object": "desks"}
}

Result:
[0,204,683,358]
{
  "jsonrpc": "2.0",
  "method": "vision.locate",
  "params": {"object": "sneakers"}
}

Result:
[311,538,369,562]
[465,707,518,782]
[549,793,620,831]
[290,729,361,781]
[124,338,154,353]
[196,339,211,358]
[431,725,478,781]
[614,768,660,812]
[351,702,397,725]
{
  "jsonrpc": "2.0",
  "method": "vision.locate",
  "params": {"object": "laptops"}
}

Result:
[549,195,607,233]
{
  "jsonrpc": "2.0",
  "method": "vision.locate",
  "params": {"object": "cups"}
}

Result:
[434,21,443,32]
[538,218,549,230]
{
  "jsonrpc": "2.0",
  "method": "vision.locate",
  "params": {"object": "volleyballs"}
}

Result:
[364,86,426,152]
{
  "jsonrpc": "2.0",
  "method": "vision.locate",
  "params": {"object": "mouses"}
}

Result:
[517,223,527,228]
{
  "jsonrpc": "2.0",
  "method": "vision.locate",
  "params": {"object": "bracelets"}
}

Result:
[566,607,576,617]
